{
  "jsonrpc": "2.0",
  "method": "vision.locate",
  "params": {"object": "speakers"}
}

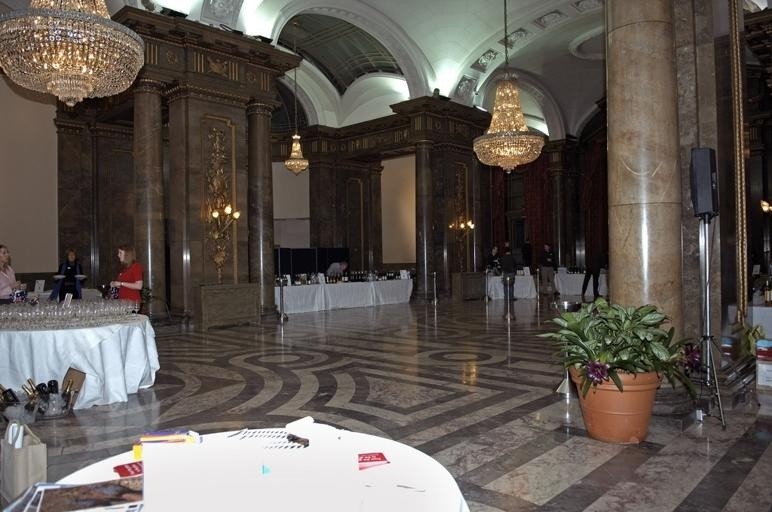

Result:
[689,147,719,220]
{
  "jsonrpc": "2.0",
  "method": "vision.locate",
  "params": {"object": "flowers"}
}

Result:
[536,297,701,402]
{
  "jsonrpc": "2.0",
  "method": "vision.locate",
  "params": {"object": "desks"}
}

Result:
[554,272,608,298]
[191,282,261,334]
[486,272,537,299]
[274,279,413,315]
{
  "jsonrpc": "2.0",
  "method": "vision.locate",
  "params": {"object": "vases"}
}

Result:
[568,365,664,445]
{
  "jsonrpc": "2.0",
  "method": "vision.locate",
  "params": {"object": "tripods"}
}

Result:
[667,224,761,430]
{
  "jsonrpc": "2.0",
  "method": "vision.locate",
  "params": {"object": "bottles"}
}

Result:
[325,269,401,283]
[567,264,586,273]
[294,273,302,285]
[306,272,312,285]
[1,376,73,414]
[515,265,524,275]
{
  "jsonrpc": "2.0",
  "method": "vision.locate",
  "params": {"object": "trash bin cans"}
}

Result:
[550,301,582,364]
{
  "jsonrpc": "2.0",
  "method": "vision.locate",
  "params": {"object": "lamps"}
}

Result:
[283,21,309,176]
[210,204,241,240]
[473,0,545,175]
[448,215,475,244]
[0,1,146,108]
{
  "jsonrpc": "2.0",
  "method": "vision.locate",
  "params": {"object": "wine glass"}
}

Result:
[0,298,141,330]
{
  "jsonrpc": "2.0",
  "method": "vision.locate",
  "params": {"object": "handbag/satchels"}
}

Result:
[0,420,48,504]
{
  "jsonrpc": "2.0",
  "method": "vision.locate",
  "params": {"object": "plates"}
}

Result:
[54,274,87,279]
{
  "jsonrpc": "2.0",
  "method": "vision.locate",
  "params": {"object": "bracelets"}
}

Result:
[120,281,124,287]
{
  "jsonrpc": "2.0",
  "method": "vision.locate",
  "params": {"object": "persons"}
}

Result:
[48,248,85,303]
[501,300,519,329]
[536,242,562,297]
[109,242,145,314]
[325,260,349,278]
[486,245,502,271]
[580,242,604,298]
[498,240,514,258]
[497,249,518,300]
[1,242,26,307]
